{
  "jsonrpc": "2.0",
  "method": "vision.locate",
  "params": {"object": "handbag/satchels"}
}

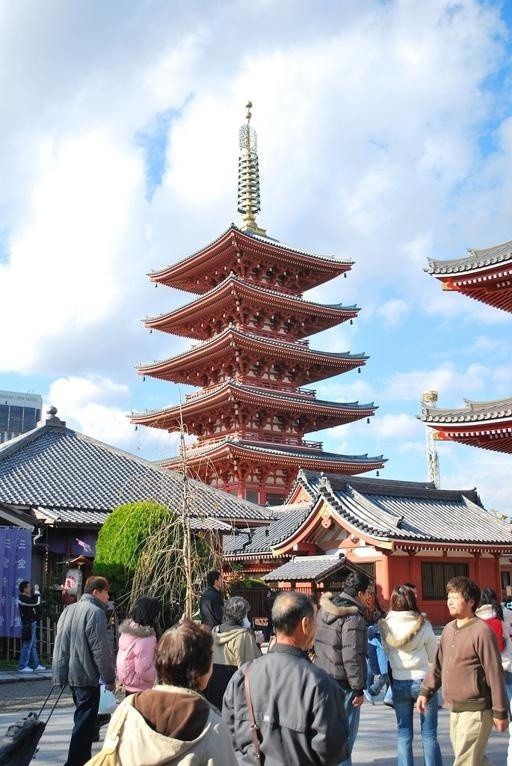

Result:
[21,624,32,640]
[84,739,121,766]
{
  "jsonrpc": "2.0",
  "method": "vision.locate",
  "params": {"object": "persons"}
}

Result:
[17,580,48,673]
[51,565,512,765]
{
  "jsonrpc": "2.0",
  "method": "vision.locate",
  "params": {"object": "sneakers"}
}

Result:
[363,690,374,705]
[383,698,394,708]
[20,667,33,672]
[35,665,46,670]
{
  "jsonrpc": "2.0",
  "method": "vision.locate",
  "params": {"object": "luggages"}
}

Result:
[1,685,64,766]
